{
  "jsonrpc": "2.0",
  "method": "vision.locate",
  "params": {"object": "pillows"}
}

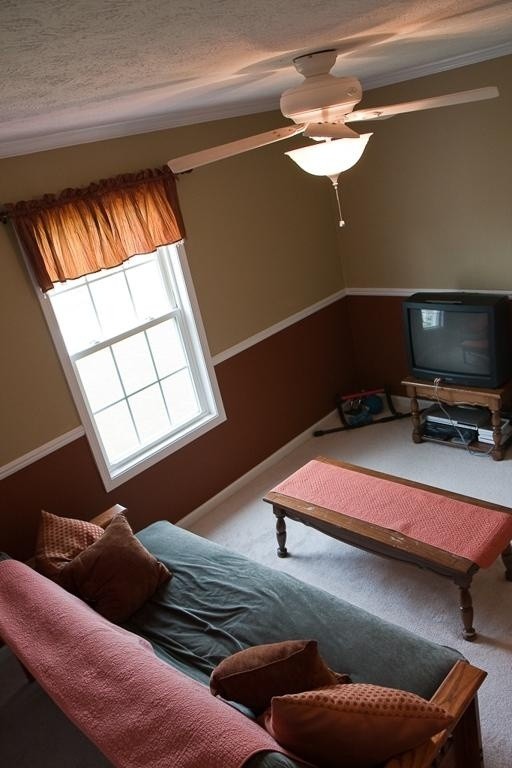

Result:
[35,507,106,577]
[209,636,352,704]
[62,515,173,626]
[257,685,459,761]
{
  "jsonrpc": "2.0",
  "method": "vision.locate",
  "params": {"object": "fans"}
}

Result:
[165,52,500,176]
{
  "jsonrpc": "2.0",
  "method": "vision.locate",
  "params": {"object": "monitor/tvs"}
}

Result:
[402,291,510,389]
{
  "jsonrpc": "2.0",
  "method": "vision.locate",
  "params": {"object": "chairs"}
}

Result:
[336,371,403,427]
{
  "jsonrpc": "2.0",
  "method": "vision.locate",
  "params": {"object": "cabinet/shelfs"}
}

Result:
[400,377,512,461]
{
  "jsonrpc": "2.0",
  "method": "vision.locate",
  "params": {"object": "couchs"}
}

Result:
[0,504,486,768]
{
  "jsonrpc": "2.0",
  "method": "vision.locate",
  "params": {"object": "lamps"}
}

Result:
[284,132,374,229]
